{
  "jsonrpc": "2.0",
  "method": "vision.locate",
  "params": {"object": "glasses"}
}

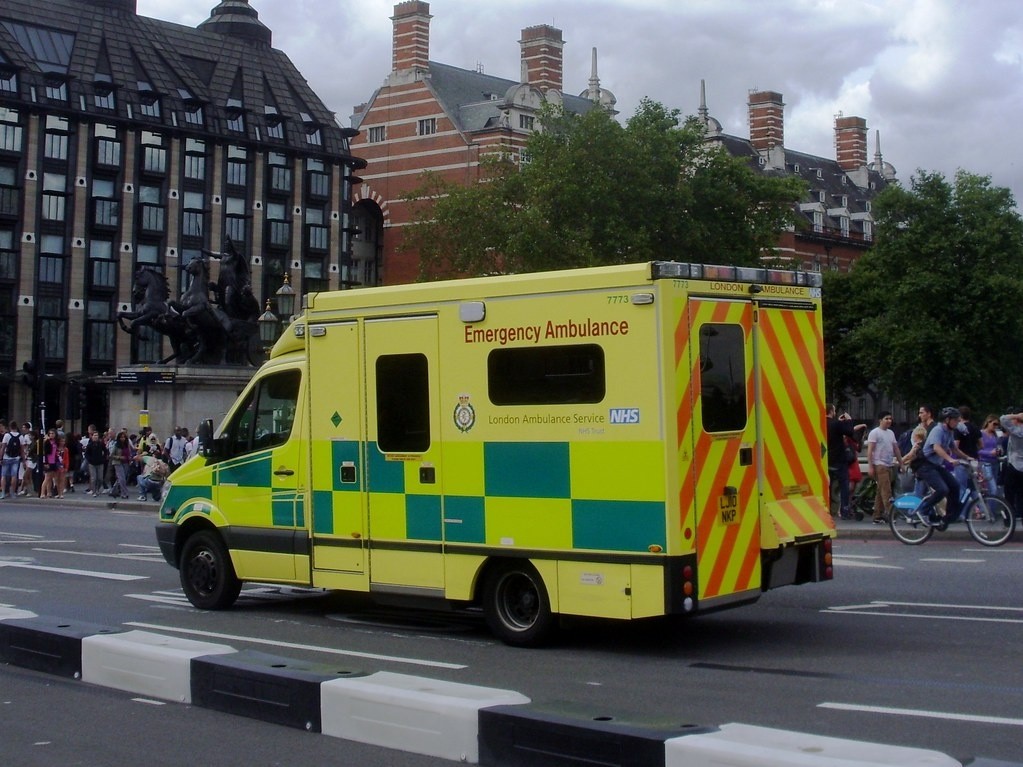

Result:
[991,421,999,427]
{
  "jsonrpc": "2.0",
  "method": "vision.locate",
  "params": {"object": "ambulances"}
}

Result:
[154,261,837,651]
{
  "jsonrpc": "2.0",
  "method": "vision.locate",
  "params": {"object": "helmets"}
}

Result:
[941,406,959,419]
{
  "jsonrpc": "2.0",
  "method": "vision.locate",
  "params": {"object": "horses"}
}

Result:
[116,257,232,365]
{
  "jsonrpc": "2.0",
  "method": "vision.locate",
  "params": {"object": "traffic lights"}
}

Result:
[72,383,87,420]
[21,361,37,388]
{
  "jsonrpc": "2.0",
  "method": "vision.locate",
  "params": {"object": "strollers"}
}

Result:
[838,463,901,522]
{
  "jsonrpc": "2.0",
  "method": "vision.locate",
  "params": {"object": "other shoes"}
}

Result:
[835,504,1023,533]
[916,510,932,528]
[0,484,147,501]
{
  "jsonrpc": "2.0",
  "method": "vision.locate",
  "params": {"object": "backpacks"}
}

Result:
[898,427,916,456]
[151,458,170,477]
[5,432,22,457]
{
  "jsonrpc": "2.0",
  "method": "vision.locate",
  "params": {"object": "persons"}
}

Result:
[255,415,270,437]
[200,236,251,314]
[827,403,1023,527]
[0,415,202,500]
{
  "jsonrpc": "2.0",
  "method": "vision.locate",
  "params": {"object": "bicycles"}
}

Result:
[890,461,1016,547]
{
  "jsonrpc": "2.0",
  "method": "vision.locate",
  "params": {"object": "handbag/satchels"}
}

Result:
[899,465,915,491]
[159,455,170,464]
[844,443,857,467]
[909,448,927,474]
